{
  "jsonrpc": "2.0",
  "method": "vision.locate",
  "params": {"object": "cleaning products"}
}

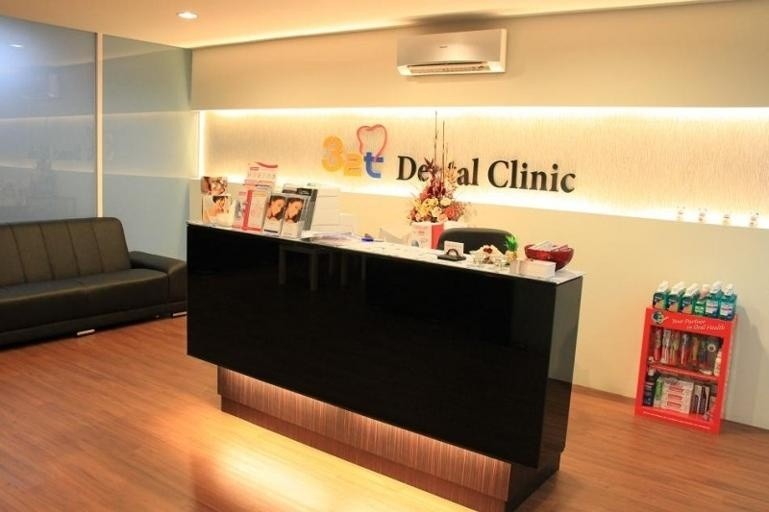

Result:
[652,281,737,321]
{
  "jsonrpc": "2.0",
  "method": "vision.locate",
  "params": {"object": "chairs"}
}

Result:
[443,229,517,260]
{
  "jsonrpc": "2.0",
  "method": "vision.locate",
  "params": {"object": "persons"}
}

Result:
[266,195,286,223]
[284,198,304,226]
[211,196,225,219]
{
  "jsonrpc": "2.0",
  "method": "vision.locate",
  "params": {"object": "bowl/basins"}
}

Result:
[524,244,573,272]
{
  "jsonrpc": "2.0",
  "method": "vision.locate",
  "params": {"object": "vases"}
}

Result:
[429,222,444,248]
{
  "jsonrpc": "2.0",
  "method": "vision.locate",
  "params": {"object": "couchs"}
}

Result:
[0,216,187,352]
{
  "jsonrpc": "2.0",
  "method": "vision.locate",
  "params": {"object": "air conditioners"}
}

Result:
[396,27,507,77]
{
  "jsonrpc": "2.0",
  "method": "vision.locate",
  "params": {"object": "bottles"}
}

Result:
[643,368,656,404]
[653,280,737,321]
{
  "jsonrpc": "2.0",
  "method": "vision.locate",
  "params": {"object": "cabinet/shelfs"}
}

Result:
[634,307,738,438]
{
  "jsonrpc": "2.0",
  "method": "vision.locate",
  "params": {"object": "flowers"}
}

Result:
[405,164,473,229]
